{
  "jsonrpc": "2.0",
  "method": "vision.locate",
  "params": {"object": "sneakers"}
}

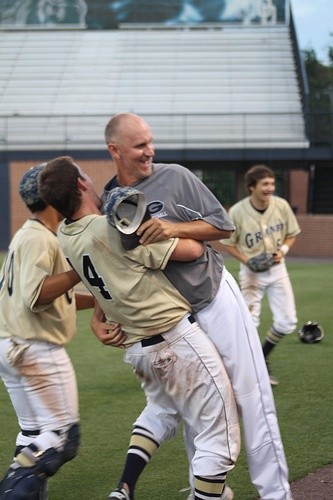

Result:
[186,486,232,500]
[266,361,279,386]
[108,488,130,500]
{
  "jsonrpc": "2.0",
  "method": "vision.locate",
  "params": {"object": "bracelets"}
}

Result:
[278,244,289,255]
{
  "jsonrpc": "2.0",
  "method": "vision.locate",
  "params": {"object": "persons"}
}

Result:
[89,114,294,500]
[37,156,241,500]
[0,163,95,500]
[220,165,301,386]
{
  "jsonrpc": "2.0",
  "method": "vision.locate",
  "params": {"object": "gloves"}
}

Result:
[5,340,28,367]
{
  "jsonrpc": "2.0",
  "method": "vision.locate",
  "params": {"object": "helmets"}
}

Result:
[298,321,325,343]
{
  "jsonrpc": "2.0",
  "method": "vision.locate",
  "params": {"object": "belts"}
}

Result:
[141,315,195,347]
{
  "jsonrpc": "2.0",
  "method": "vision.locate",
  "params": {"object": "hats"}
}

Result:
[103,186,153,250]
[18,162,48,205]
